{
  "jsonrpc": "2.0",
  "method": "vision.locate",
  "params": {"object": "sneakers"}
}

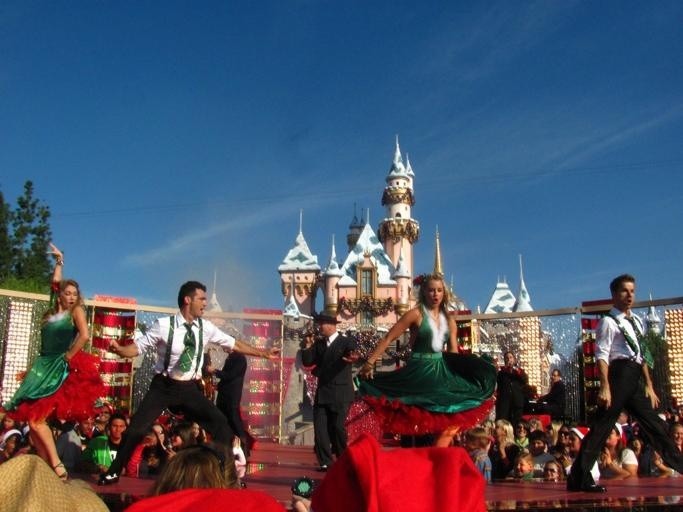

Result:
[96,470,120,486]
[242,435,255,458]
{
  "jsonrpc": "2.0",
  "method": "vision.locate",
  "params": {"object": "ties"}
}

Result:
[624,316,654,371]
[174,322,196,373]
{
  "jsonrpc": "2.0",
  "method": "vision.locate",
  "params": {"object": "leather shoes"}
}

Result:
[312,463,330,473]
[568,473,608,493]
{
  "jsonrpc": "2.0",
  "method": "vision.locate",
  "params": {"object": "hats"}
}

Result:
[569,424,589,439]
[312,309,342,324]
[0,426,22,451]
[0,455,110,511]
[615,423,629,446]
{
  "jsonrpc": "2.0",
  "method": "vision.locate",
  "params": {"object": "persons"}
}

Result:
[297,272,499,450]
[0,240,313,512]
[455,353,682,482]
[566,274,682,493]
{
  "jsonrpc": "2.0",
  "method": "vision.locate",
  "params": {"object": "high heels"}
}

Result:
[51,460,68,483]
